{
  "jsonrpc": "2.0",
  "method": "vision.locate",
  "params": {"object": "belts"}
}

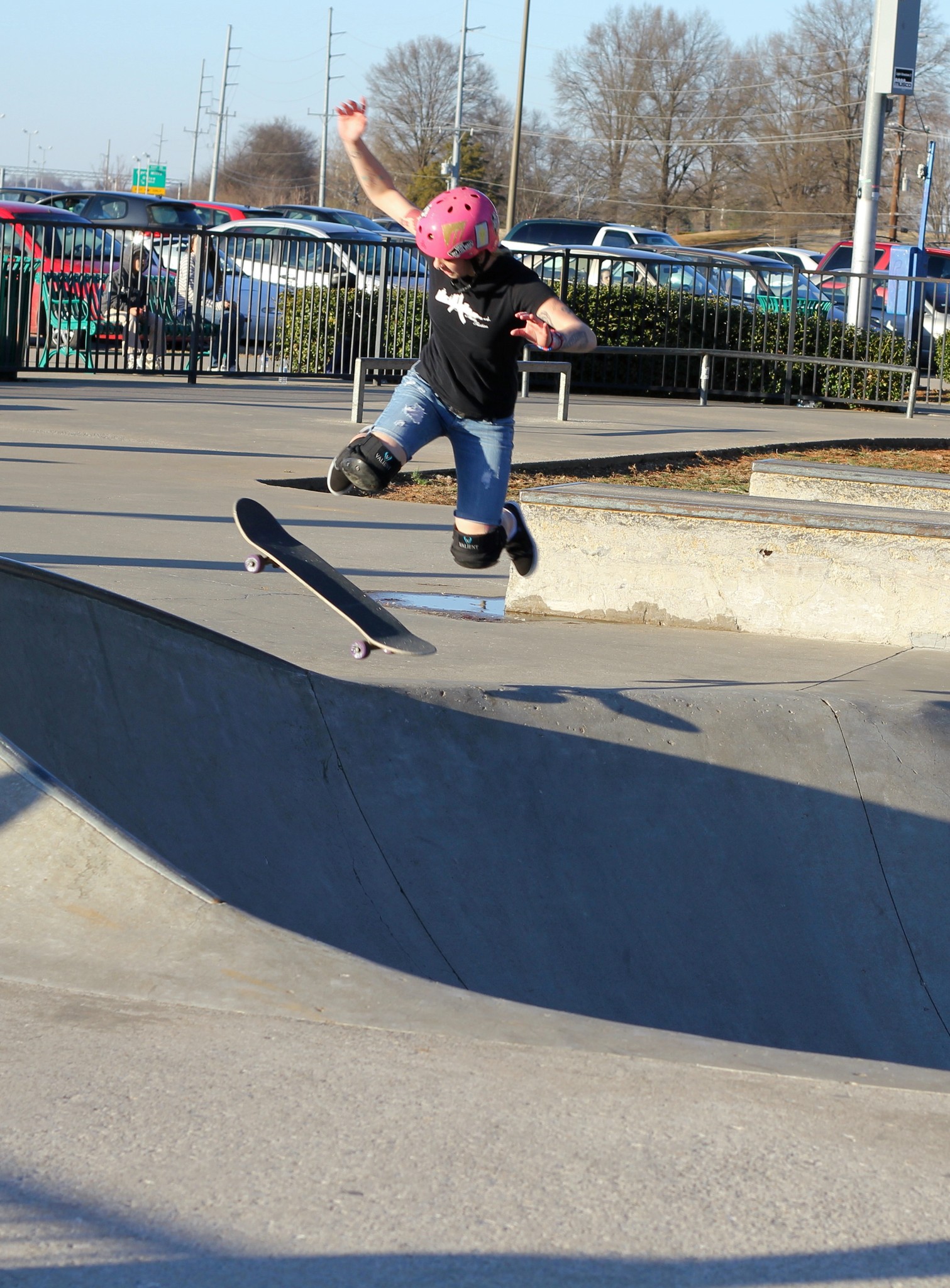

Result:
[444,403,486,420]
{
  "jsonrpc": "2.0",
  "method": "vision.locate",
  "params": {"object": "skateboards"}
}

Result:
[232,498,438,660]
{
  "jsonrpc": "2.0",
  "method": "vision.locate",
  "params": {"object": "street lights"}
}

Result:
[132,155,141,194]
[21,128,39,187]
[142,152,151,195]
[36,144,52,188]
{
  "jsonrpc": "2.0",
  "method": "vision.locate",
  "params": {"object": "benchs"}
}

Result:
[33,271,249,375]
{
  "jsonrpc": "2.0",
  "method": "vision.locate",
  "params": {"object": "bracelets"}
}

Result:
[553,331,563,351]
[536,327,555,351]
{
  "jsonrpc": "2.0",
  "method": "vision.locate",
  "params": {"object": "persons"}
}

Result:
[329,96,595,578]
[171,234,244,371]
[100,243,167,370]
[600,268,614,285]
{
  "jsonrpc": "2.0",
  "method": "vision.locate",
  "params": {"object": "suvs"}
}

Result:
[501,216,682,253]
[810,240,950,313]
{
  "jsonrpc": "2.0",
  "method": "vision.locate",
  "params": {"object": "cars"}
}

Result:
[0,182,431,269]
[712,246,827,295]
[517,245,754,324]
[143,218,429,351]
[628,243,895,336]
[749,268,950,373]
[0,200,217,351]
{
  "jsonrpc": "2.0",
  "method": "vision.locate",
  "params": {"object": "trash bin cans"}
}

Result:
[756,295,837,320]
[0,256,46,380]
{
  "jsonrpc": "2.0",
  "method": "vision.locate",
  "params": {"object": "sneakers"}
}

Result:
[328,443,354,496]
[503,500,534,578]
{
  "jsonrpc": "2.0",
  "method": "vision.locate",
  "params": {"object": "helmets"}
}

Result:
[415,186,500,261]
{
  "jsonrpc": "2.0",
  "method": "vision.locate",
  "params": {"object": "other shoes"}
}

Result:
[145,357,160,370]
[229,365,236,372]
[124,358,139,369]
[206,366,225,372]
